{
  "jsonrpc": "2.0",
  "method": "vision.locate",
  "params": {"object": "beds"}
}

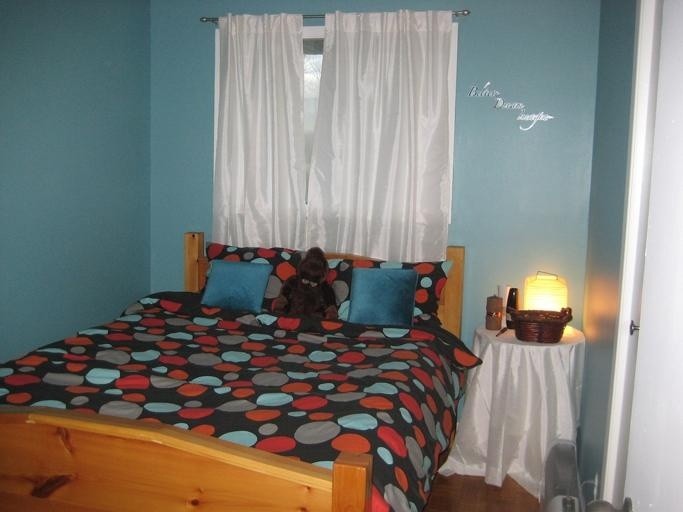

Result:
[0,232,465,512]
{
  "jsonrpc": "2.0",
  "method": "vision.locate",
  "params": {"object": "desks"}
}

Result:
[436,324,586,502]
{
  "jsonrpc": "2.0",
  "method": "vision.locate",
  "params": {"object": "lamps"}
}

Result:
[523,271,569,312]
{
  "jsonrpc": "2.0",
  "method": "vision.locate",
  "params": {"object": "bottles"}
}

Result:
[485,286,518,329]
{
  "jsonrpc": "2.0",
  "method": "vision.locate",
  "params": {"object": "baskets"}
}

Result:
[507,307,573,343]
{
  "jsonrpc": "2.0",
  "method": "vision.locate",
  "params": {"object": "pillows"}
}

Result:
[324,258,454,330]
[199,259,274,314]
[346,266,419,326]
[204,240,299,313]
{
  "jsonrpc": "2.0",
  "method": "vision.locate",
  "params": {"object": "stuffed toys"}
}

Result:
[274,245,339,322]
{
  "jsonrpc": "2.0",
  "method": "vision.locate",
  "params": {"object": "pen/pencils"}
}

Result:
[496,328,508,337]
